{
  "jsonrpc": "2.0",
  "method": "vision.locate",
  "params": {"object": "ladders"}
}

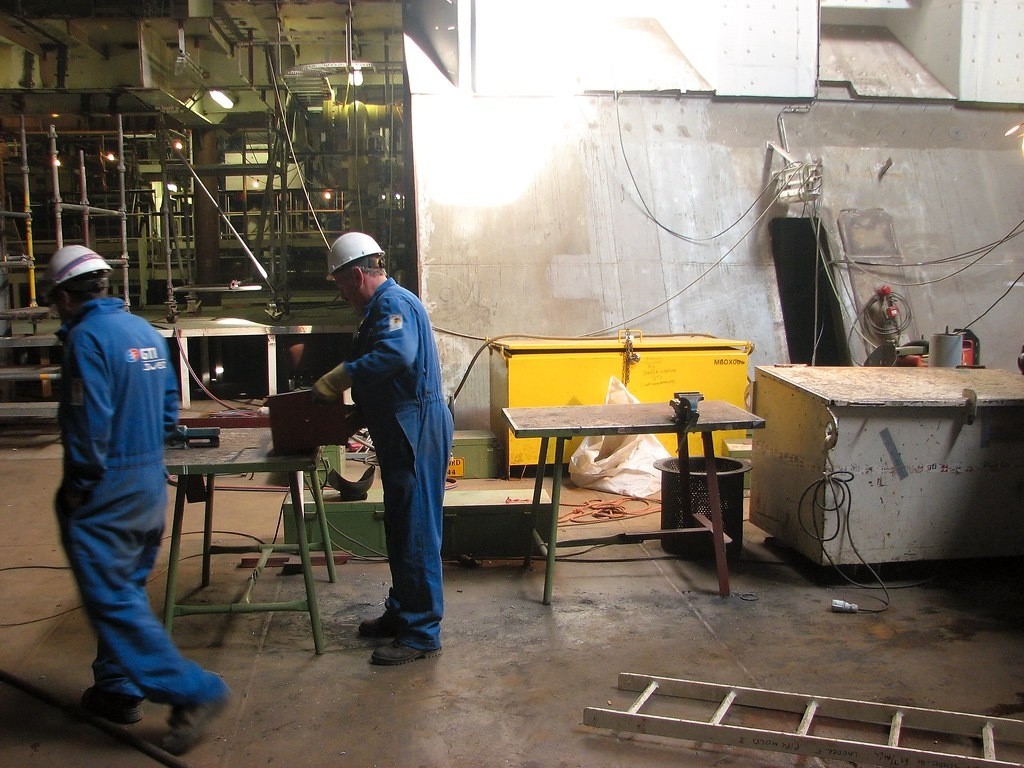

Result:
[580,670,1024,768]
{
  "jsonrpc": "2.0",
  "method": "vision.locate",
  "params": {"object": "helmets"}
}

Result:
[41,243,112,293]
[328,231,386,273]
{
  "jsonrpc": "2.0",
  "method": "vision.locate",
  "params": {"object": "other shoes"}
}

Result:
[371,637,444,665]
[160,685,235,755]
[80,686,146,724]
[358,611,405,637]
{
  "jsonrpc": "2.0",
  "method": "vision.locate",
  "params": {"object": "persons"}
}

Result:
[314,230,452,666]
[47,242,233,757]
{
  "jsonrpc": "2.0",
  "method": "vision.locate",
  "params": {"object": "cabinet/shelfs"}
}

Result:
[284,489,553,559]
[303,445,344,489]
[447,429,502,479]
[748,363,1024,578]
[723,439,752,489]
[491,340,755,479]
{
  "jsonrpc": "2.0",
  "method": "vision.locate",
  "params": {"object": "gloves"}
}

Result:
[313,362,353,409]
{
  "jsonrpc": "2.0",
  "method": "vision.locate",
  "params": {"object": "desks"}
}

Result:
[163,427,336,654]
[501,400,766,606]
